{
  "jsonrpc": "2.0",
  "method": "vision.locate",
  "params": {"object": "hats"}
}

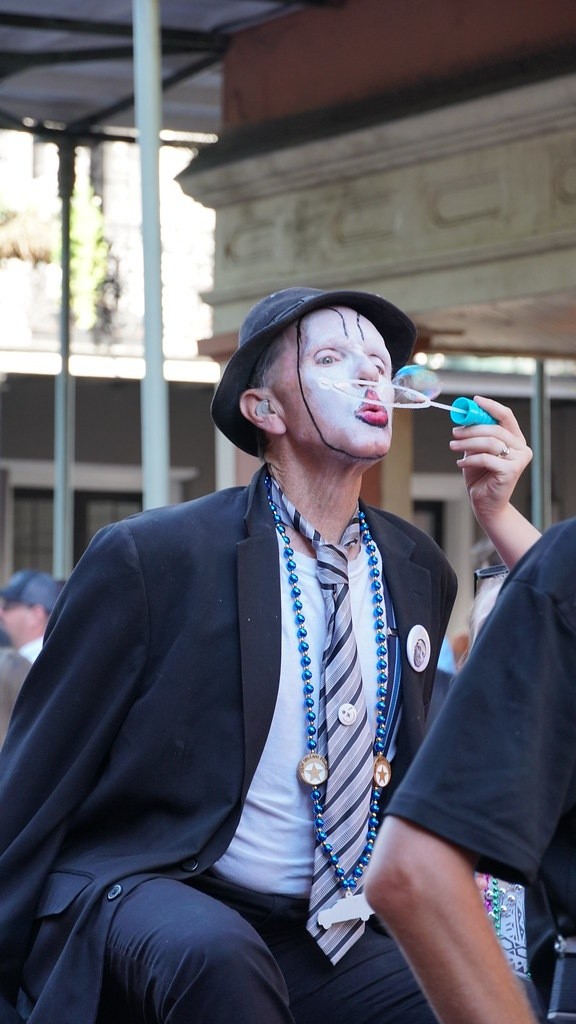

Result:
[0,567,59,615]
[210,286,418,459]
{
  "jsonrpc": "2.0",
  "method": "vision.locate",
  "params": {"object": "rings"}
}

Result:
[496,447,511,459]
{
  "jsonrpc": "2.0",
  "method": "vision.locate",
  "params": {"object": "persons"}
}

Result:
[365,517,575,1024]
[0,568,60,744]
[448,394,545,978]
[2,284,457,1024]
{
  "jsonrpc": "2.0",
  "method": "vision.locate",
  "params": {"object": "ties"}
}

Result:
[269,478,372,967]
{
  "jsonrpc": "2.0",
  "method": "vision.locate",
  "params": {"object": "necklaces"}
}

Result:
[474,872,501,940]
[263,475,390,931]
